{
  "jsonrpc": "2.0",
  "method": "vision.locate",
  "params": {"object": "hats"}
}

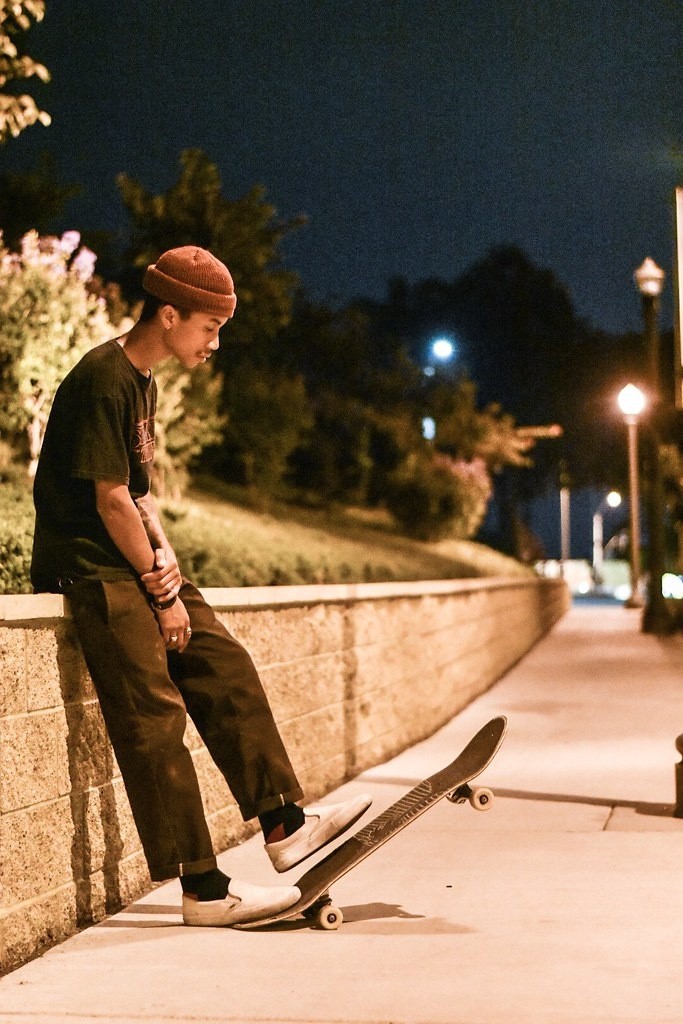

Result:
[142,245,237,317]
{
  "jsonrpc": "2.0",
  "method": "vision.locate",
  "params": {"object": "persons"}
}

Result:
[29,246,374,925]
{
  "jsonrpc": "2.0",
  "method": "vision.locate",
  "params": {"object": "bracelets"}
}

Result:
[149,591,176,610]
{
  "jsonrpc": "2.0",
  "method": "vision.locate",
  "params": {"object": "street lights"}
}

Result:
[615,380,649,609]
[592,491,623,589]
[635,253,677,635]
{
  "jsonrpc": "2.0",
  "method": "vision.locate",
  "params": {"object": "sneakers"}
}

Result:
[182,878,301,926]
[264,795,371,873]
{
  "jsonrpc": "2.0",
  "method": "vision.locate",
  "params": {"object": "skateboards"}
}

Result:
[229,714,509,931]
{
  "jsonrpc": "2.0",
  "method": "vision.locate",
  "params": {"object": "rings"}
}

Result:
[185,627,191,634]
[170,636,178,641]
